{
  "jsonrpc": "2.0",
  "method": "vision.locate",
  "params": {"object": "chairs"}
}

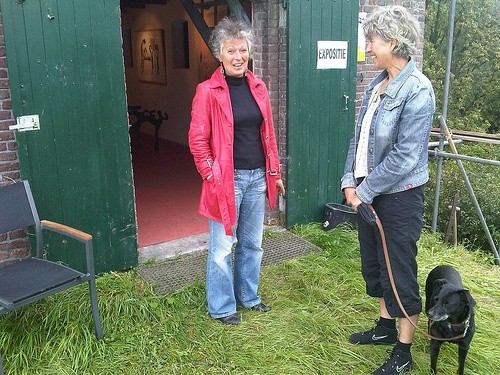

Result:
[0,180,104,340]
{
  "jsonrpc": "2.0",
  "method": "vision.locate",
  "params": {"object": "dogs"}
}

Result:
[425,265,477,375]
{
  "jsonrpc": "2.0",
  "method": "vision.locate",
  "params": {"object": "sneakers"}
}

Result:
[371,348,413,375]
[348,319,398,345]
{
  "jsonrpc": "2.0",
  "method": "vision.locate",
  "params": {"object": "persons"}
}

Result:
[188,17,285,325]
[338,4,437,375]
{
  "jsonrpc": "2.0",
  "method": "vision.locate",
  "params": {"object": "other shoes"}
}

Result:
[218,313,243,324]
[248,302,271,314]
